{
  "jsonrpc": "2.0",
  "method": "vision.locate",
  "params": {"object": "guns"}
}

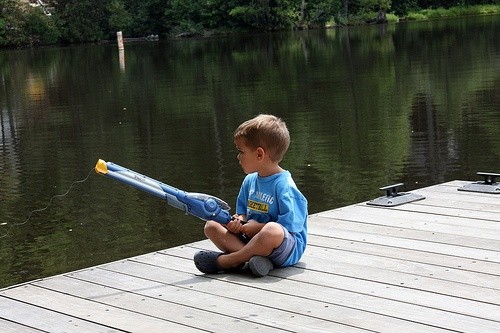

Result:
[94,157,250,231]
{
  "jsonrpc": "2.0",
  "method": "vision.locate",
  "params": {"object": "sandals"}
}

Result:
[249,255,274,277]
[194,250,232,274]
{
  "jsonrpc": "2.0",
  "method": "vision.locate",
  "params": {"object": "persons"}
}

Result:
[194,115,308,277]
